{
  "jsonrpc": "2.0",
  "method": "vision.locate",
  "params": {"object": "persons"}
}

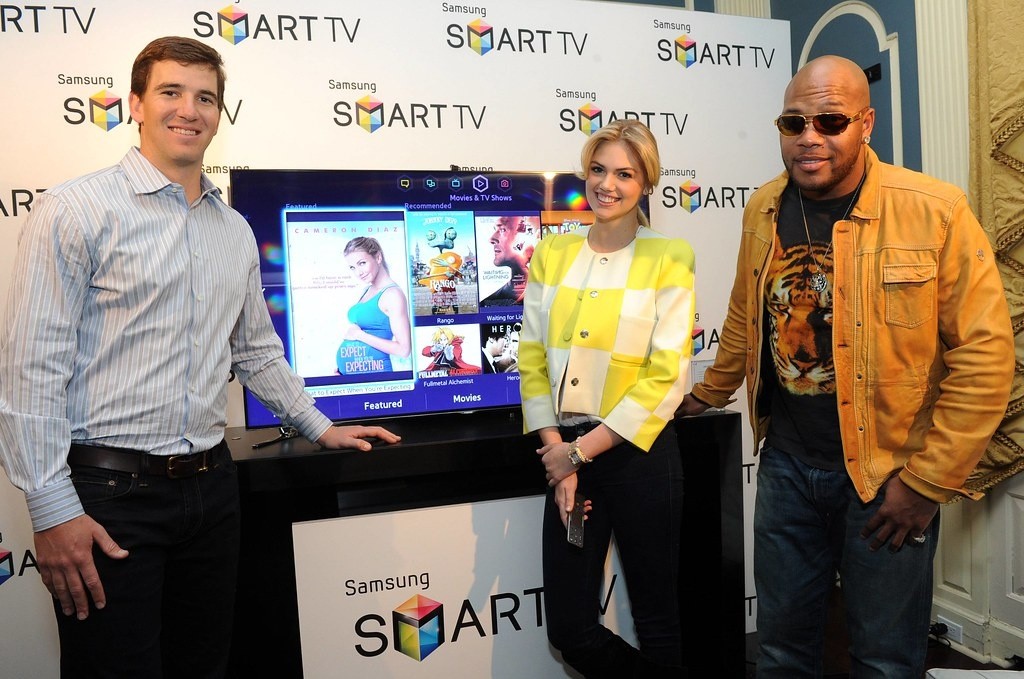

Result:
[479,216,540,307]
[0,34,401,679]
[482,324,511,374]
[674,54,1016,679]
[332,236,410,375]
[518,119,695,679]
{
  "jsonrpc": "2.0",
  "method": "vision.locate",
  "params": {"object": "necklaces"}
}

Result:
[798,172,866,292]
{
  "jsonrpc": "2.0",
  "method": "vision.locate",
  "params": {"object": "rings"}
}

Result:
[911,534,926,544]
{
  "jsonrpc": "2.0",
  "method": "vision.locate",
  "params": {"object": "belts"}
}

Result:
[67,437,226,478]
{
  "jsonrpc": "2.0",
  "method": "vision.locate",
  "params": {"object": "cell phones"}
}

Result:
[567,498,585,548]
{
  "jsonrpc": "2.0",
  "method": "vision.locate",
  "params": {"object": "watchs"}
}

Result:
[568,436,593,466]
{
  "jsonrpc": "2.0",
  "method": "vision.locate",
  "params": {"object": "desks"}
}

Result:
[222,408,751,679]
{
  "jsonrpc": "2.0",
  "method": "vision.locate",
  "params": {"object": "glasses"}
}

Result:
[773,106,870,138]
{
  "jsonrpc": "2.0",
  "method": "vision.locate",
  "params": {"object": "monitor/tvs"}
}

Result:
[227,169,658,448]
